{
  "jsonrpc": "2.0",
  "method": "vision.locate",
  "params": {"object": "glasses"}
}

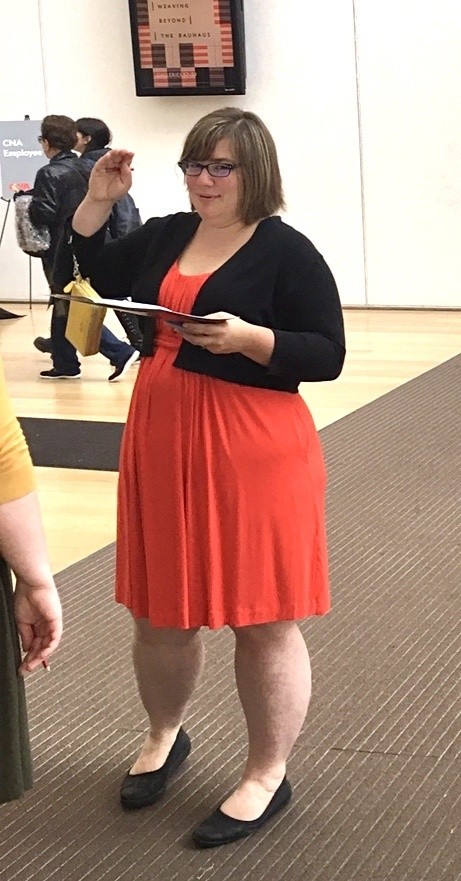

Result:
[177,159,241,178]
[38,135,46,144]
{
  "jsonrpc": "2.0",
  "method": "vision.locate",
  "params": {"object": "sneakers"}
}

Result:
[39,363,81,379]
[108,348,141,381]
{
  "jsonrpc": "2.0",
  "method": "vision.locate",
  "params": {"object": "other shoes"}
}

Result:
[34,335,55,353]
[117,723,192,809]
[192,776,292,846]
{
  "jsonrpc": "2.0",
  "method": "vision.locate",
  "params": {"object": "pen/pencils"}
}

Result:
[99,167,134,173]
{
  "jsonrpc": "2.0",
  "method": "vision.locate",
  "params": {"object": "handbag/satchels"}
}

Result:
[63,272,107,357]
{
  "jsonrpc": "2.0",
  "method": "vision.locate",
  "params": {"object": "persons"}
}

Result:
[71,107,346,847]
[0,358,63,804]
[14,115,145,382]
